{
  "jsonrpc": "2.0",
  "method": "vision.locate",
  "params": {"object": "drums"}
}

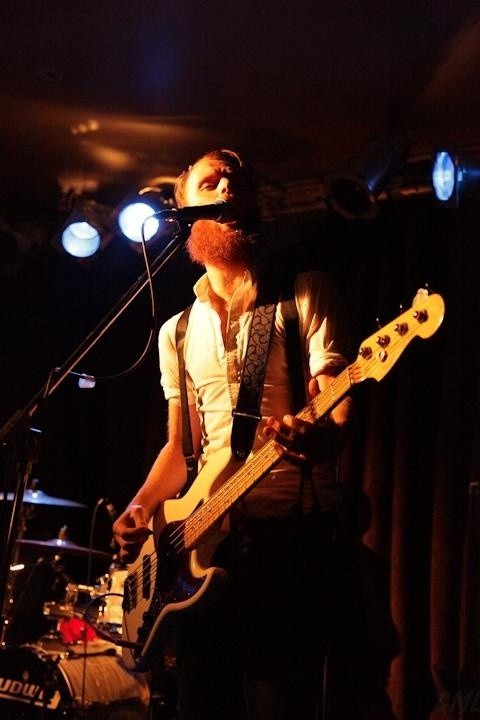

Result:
[0,635,152,720]
[64,583,104,616]
[98,564,130,625]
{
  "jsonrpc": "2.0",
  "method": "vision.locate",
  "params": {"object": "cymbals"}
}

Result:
[15,538,112,560]
[2,491,85,510]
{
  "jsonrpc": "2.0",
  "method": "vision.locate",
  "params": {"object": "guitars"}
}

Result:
[121,285,446,668]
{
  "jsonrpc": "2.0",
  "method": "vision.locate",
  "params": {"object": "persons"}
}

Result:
[113,148,365,716]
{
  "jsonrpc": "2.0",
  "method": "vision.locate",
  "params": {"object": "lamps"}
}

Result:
[324,149,400,219]
[117,190,166,243]
[429,145,479,203]
[57,199,113,258]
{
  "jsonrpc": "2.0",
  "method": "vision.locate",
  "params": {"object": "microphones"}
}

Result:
[156,200,236,226]
